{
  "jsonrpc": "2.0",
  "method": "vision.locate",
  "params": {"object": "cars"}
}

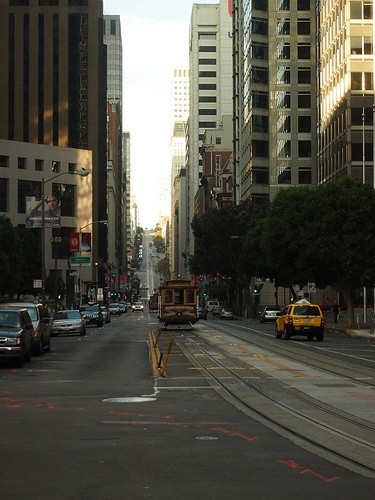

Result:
[196,306,208,321]
[50,309,87,337]
[125,302,131,309]
[119,304,127,313]
[257,305,282,324]
[100,306,112,324]
[218,307,235,320]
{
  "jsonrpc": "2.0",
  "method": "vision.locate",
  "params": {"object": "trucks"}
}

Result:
[206,300,220,312]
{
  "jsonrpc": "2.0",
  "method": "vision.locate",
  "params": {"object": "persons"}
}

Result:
[332,301,340,323]
[30,196,60,217]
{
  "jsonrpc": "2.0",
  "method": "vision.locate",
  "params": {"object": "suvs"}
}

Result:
[274,296,325,342]
[109,303,121,316]
[131,302,144,313]
[78,304,104,328]
[0,307,35,368]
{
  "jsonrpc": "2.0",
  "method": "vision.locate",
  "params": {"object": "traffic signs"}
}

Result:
[70,256,91,264]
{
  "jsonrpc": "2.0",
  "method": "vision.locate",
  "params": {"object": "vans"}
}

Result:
[0,302,53,357]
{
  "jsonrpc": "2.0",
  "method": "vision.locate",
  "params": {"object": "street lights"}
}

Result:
[78,219,110,292]
[40,167,91,304]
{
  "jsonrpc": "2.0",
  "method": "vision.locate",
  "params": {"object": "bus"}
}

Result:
[158,278,199,331]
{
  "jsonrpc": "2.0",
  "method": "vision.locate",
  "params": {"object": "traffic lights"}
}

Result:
[253,284,258,294]
[114,291,117,300]
